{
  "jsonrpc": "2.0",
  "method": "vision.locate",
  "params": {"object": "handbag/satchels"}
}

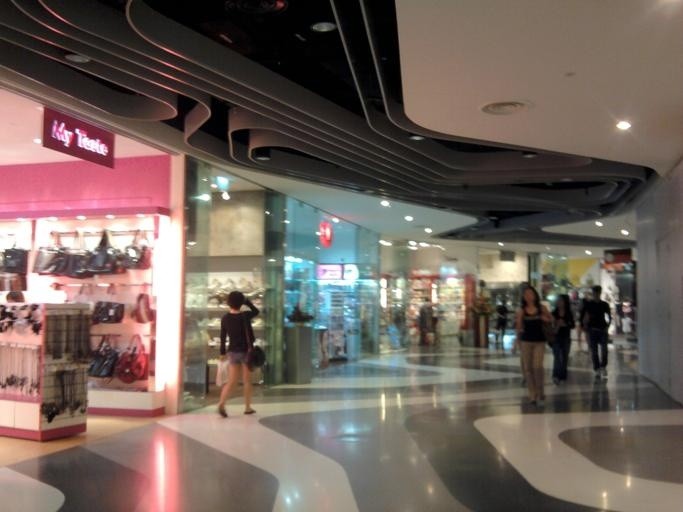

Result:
[87,346,148,384]
[543,325,556,347]
[32,245,151,279]
[215,360,229,387]
[248,345,266,367]
[0,249,27,291]
[91,293,155,324]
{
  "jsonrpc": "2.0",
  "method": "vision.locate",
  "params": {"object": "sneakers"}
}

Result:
[552,378,564,387]
[592,367,607,378]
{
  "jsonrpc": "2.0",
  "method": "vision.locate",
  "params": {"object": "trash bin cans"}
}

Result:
[286,323,313,385]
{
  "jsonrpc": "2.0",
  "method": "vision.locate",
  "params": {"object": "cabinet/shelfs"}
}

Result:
[0,299,94,441]
[50,228,154,381]
[207,284,266,370]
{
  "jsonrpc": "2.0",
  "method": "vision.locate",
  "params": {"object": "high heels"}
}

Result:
[528,400,546,409]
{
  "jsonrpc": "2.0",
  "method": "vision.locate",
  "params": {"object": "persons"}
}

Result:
[214,290,266,417]
[547,294,575,387]
[418,296,432,345]
[510,340,527,385]
[495,299,508,344]
[515,284,553,409]
[589,374,613,454]
[318,330,328,369]
[579,285,611,378]
[577,290,590,351]
[614,293,624,334]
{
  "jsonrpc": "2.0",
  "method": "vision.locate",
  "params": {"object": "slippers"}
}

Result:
[217,407,227,417]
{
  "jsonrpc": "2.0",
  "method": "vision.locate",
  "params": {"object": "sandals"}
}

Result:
[243,409,257,415]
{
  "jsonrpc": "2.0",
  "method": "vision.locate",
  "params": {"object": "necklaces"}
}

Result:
[0,312,93,424]
